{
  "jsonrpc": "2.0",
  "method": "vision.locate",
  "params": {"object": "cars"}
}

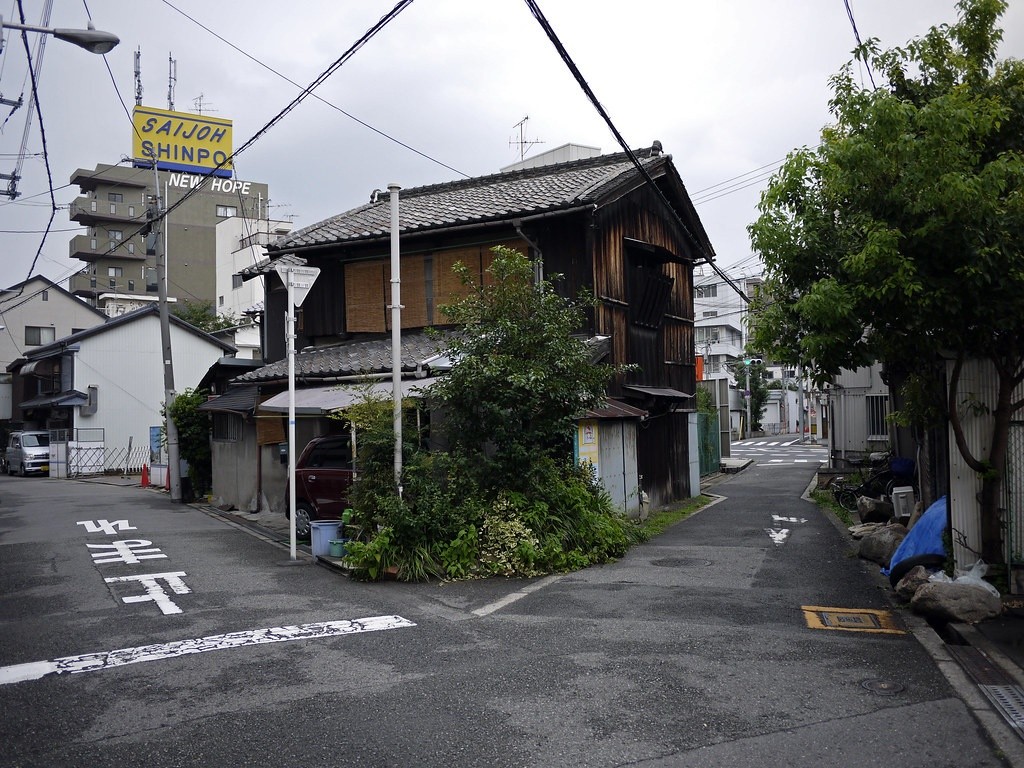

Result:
[285,436,362,538]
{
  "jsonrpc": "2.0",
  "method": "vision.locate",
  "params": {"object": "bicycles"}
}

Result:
[837,447,920,511]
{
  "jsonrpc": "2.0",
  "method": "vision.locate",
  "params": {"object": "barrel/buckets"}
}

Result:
[310,520,342,556]
[328,539,344,558]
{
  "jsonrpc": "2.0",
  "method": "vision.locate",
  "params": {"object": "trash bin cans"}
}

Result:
[308,520,342,557]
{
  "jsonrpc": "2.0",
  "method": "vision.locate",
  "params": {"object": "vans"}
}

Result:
[5,431,53,477]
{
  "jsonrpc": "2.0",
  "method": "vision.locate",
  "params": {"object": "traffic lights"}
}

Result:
[744,358,763,366]
